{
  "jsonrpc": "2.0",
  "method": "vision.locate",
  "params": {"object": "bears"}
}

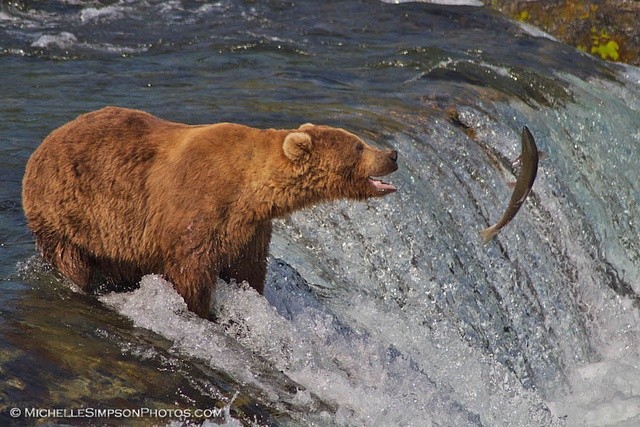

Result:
[22,104,398,322]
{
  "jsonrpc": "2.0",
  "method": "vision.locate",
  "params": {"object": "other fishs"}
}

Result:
[480,126,546,240]
